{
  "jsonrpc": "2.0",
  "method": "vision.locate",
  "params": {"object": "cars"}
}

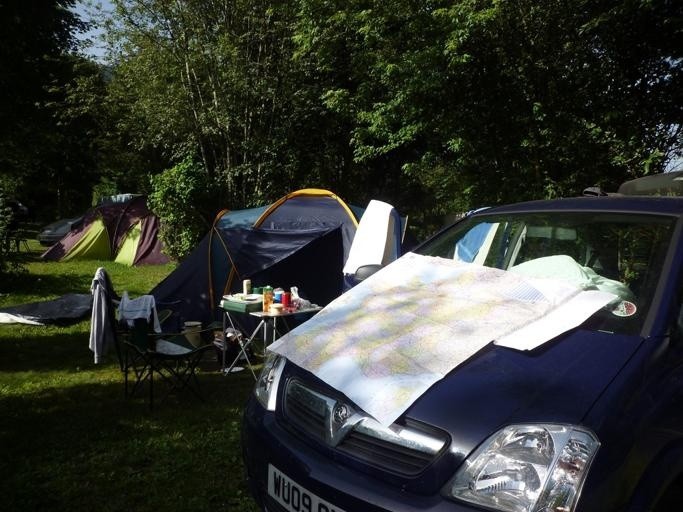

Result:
[37,217,83,248]
[241,194,683,510]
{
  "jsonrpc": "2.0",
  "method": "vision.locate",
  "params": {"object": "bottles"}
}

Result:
[242,279,293,315]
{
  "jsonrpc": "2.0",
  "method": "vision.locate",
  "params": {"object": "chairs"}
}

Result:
[91,266,221,412]
[586,249,619,282]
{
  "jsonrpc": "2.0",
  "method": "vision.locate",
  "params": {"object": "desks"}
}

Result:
[220,291,322,383]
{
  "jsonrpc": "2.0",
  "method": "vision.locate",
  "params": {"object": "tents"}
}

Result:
[133,188,420,346]
[39,193,170,268]
